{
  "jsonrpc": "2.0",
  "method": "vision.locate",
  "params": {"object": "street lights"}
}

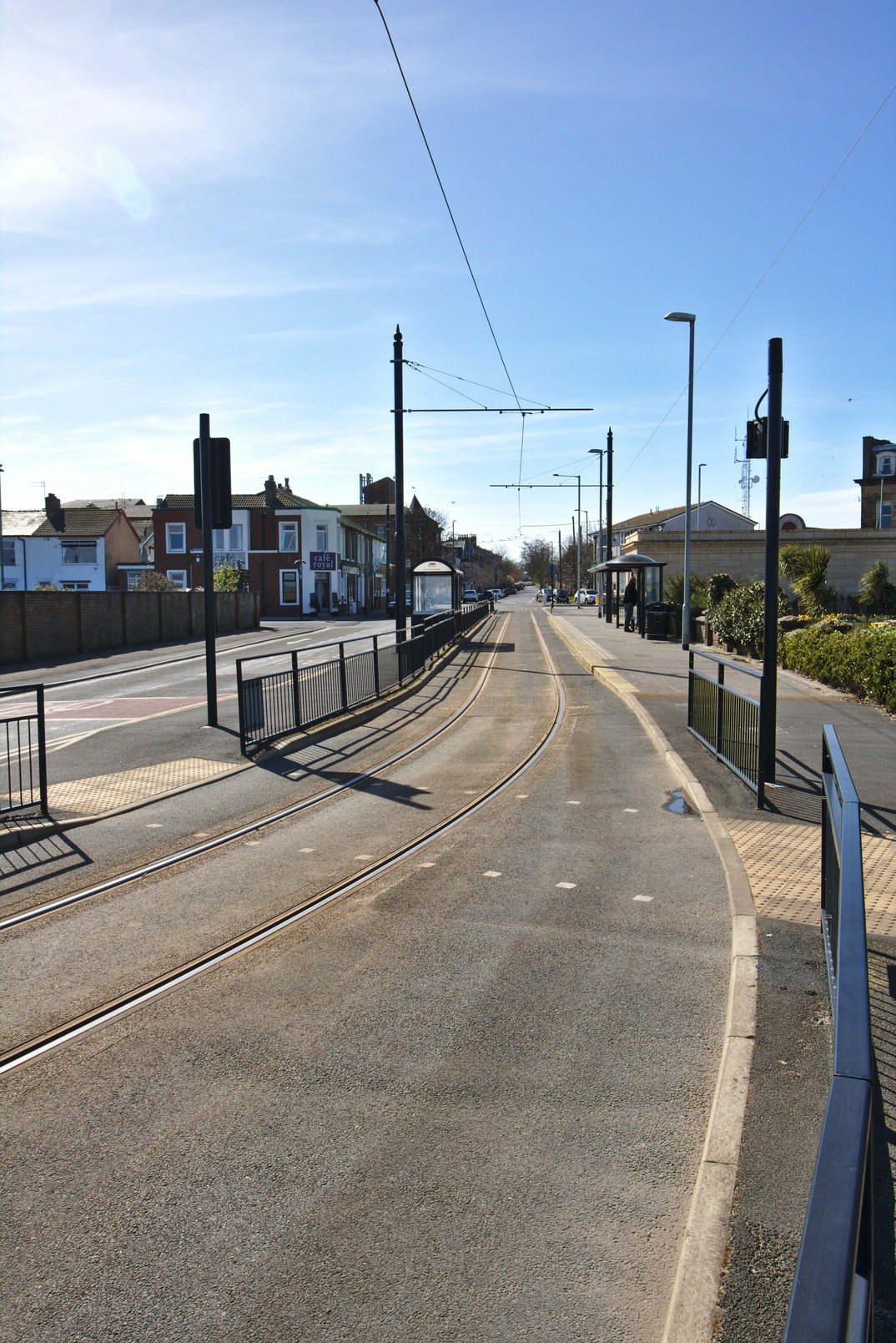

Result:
[453,519,456,564]
[587,448,604,618]
[663,311,695,651]
[575,509,588,544]
[553,473,581,609]
[697,464,707,530]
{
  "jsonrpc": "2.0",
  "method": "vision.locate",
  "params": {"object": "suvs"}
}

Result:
[462,589,480,602]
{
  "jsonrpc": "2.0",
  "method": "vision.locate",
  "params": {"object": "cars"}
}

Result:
[388,589,425,616]
[478,581,532,603]
[574,589,599,605]
[536,587,569,603]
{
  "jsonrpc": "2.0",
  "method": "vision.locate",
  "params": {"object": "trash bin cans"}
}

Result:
[643,600,668,640]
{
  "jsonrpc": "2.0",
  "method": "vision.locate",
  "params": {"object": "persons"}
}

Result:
[622,577,638,632]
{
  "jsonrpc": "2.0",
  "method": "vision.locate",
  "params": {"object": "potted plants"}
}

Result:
[332,599,340,617]
[310,601,320,617]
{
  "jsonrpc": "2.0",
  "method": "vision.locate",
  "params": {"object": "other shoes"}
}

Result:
[624,629,633,632]
